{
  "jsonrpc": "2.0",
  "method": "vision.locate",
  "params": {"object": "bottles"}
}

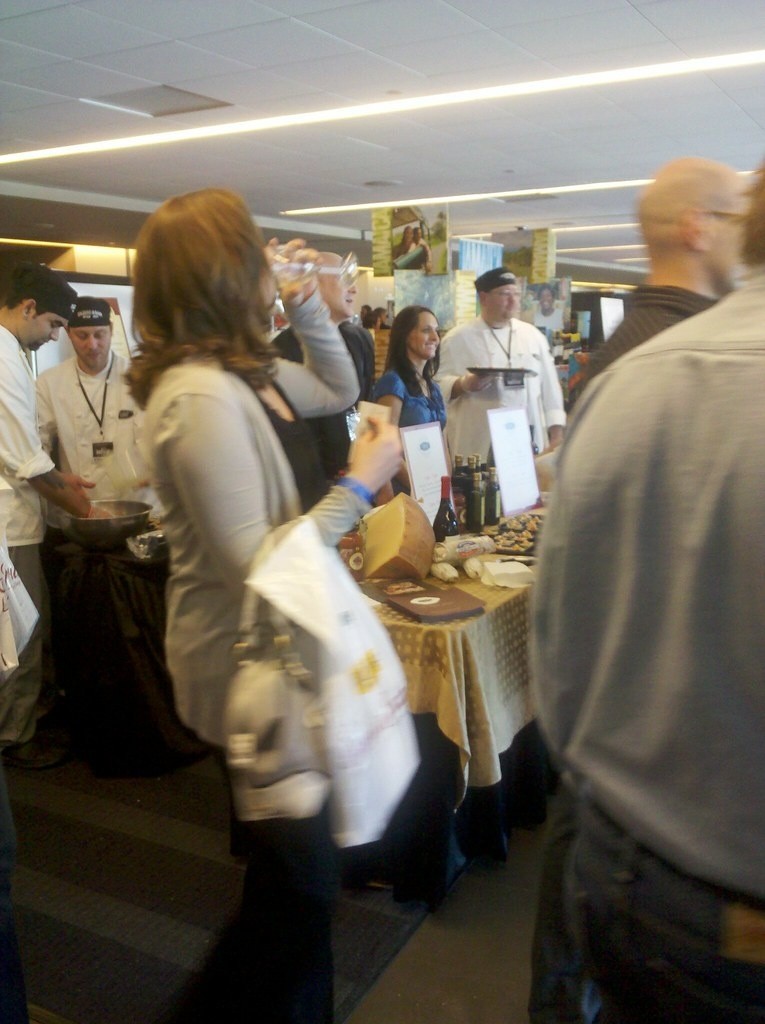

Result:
[338,524,365,581]
[433,454,502,542]
[529,425,538,455]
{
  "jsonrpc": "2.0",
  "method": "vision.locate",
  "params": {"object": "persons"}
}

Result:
[125,185,409,1024]
[270,250,566,583]
[0,261,203,1024]
[398,226,432,271]
[526,284,565,330]
[527,154,765,1024]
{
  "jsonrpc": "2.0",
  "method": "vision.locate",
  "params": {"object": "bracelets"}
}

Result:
[338,478,376,505]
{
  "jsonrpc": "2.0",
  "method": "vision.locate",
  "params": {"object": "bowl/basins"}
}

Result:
[64,501,153,539]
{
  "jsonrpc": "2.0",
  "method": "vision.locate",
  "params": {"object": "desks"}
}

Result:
[356,506,549,912]
[34,540,211,777]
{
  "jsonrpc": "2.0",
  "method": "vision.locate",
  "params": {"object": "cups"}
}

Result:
[99,447,137,489]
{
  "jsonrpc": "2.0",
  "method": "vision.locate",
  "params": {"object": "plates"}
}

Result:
[467,366,537,379]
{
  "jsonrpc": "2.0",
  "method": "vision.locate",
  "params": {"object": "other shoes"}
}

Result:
[0,733,64,768]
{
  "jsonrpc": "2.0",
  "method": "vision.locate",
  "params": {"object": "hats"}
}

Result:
[473,266,516,294]
[69,296,111,327]
[8,260,78,321]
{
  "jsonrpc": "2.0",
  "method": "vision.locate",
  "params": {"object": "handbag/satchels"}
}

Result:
[222,526,330,822]
[243,514,422,847]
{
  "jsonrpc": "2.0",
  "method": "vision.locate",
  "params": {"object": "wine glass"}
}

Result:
[266,245,358,289]
